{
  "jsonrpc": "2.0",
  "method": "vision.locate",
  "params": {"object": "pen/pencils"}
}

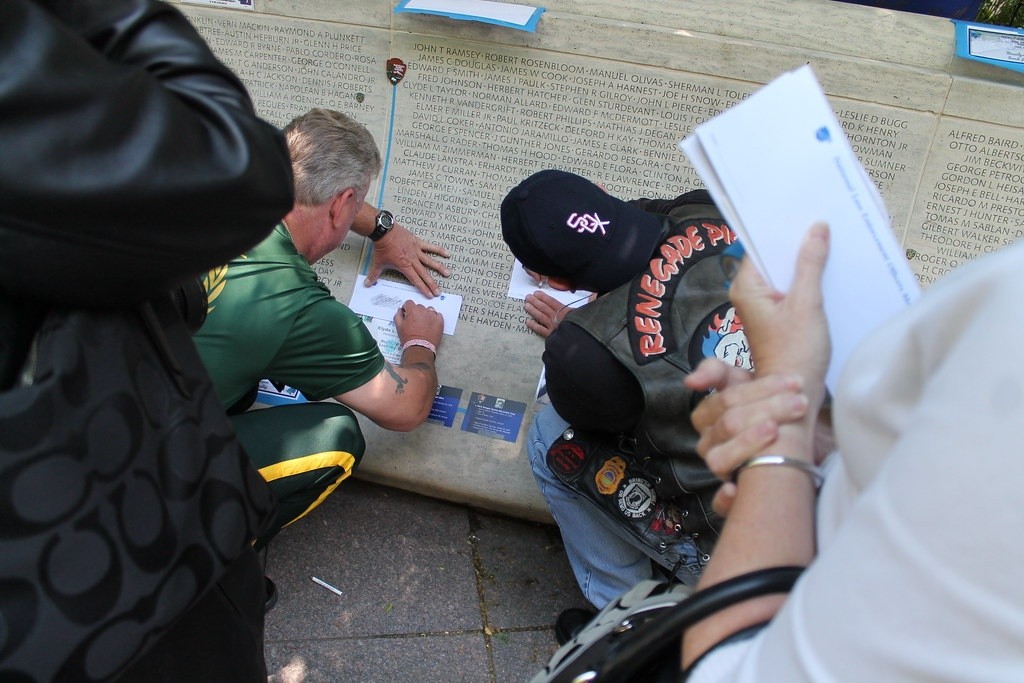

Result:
[538,280,543,289]
[398,304,402,308]
[308,573,344,596]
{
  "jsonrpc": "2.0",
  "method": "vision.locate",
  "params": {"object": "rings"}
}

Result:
[429,308,437,313]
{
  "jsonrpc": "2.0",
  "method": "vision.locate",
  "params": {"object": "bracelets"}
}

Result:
[402,338,438,360]
[733,455,825,489]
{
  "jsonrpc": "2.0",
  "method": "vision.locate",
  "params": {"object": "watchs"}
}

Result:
[367,208,393,242]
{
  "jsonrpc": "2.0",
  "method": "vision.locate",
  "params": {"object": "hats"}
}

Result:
[500,169,662,292]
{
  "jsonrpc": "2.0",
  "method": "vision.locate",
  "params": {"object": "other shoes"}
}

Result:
[553,604,593,646]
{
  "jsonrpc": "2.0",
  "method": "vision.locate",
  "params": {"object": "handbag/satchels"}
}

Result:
[0,280,275,683]
[523,566,804,683]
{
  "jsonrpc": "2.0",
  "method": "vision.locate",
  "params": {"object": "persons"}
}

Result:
[0,3,296,683]
[192,108,452,551]
[498,169,755,647]
[686,220,1024,683]
